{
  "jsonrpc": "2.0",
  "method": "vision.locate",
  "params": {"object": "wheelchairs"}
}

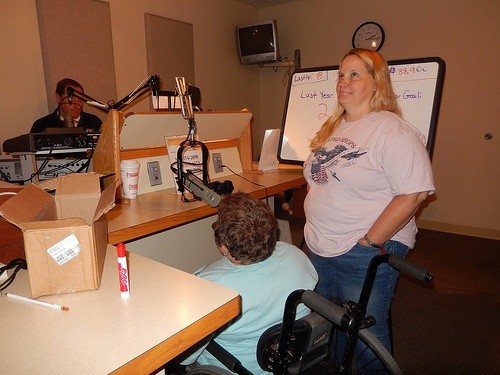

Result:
[178,252,436,375]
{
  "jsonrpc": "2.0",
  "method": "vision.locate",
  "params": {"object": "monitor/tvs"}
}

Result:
[236,19,280,65]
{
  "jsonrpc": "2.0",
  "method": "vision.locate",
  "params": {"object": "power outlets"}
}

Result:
[212,152,225,173]
[147,161,162,185]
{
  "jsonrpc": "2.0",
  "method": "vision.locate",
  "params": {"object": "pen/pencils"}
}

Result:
[6,293,69,313]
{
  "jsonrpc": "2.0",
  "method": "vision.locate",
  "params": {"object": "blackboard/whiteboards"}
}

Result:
[279,61,440,171]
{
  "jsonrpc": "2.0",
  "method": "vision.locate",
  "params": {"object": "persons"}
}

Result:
[303,48,436,375]
[30,78,103,132]
[181,194,319,375]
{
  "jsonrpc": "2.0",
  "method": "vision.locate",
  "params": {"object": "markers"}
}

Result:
[117,243,130,308]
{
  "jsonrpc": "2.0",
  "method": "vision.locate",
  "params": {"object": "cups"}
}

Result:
[120,159,140,199]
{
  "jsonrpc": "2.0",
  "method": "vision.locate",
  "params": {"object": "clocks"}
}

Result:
[352,21,385,52]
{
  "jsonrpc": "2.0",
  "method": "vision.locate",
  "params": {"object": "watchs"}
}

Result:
[364,234,384,248]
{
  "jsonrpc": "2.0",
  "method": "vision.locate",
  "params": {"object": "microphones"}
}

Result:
[60,109,75,128]
[177,169,221,208]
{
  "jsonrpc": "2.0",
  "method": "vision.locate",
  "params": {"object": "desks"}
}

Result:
[1,160,309,373]
